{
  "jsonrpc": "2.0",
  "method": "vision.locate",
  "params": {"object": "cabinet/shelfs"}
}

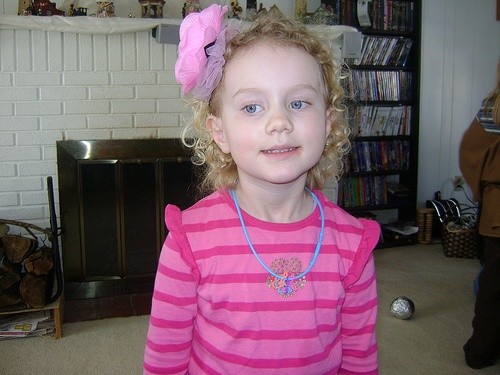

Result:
[294,0,423,250]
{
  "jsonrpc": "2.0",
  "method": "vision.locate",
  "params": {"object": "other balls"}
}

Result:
[391,297,414,319]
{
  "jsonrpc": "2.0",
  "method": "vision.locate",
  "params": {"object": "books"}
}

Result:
[335,174,411,209]
[0,309,56,342]
[341,138,416,175]
[340,69,415,104]
[346,105,413,139]
[342,36,415,68]
[350,1,417,37]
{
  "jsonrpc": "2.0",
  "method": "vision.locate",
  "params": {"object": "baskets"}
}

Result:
[441,220,477,258]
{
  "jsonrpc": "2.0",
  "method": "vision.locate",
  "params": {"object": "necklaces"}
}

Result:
[231,186,325,297]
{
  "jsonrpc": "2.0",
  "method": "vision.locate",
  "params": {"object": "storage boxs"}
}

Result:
[442,217,478,259]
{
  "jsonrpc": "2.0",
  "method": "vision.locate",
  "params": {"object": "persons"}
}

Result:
[140,12,381,375]
[459,57,500,370]
